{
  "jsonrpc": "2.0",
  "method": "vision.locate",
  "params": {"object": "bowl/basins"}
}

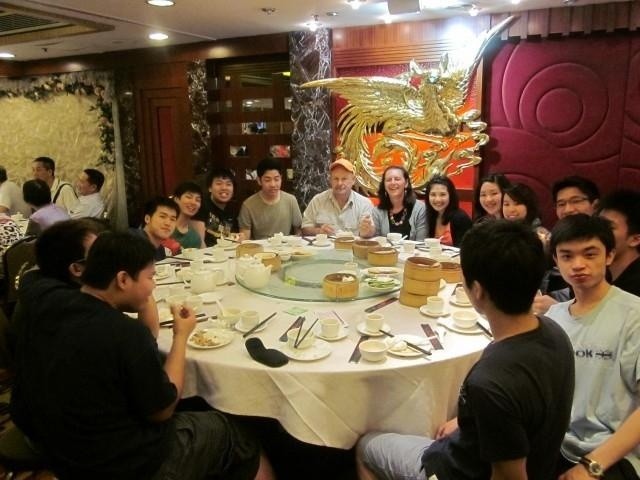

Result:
[453,310,479,328]
[287,329,314,350]
[217,308,241,325]
[359,340,388,362]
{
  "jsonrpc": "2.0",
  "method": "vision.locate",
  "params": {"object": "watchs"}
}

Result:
[580,457,604,476]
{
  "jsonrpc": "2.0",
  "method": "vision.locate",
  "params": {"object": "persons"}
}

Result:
[357,220,575,480]
[1,157,105,235]
[543,176,640,302]
[544,214,640,480]
[474,174,551,259]
[12,217,261,480]
[361,166,425,241]
[426,175,472,246]
[139,156,303,260]
[304,158,375,236]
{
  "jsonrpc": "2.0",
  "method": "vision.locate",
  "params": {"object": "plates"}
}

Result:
[315,327,348,340]
[235,319,266,332]
[279,339,332,361]
[159,313,209,328]
[151,230,474,318]
[384,334,432,356]
[186,329,233,348]
[356,321,392,337]
[444,315,490,334]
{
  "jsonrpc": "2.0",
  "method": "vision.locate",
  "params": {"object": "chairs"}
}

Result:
[0,212,113,480]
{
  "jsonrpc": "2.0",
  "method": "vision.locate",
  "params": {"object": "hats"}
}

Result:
[329,158,355,173]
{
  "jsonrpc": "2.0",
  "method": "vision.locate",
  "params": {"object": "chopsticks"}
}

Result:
[243,312,278,337]
[477,321,493,337]
[379,331,432,356]
[294,318,319,347]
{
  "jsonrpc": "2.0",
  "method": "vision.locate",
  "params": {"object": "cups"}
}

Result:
[367,314,384,333]
[10,213,23,224]
[242,310,259,329]
[320,318,339,337]
